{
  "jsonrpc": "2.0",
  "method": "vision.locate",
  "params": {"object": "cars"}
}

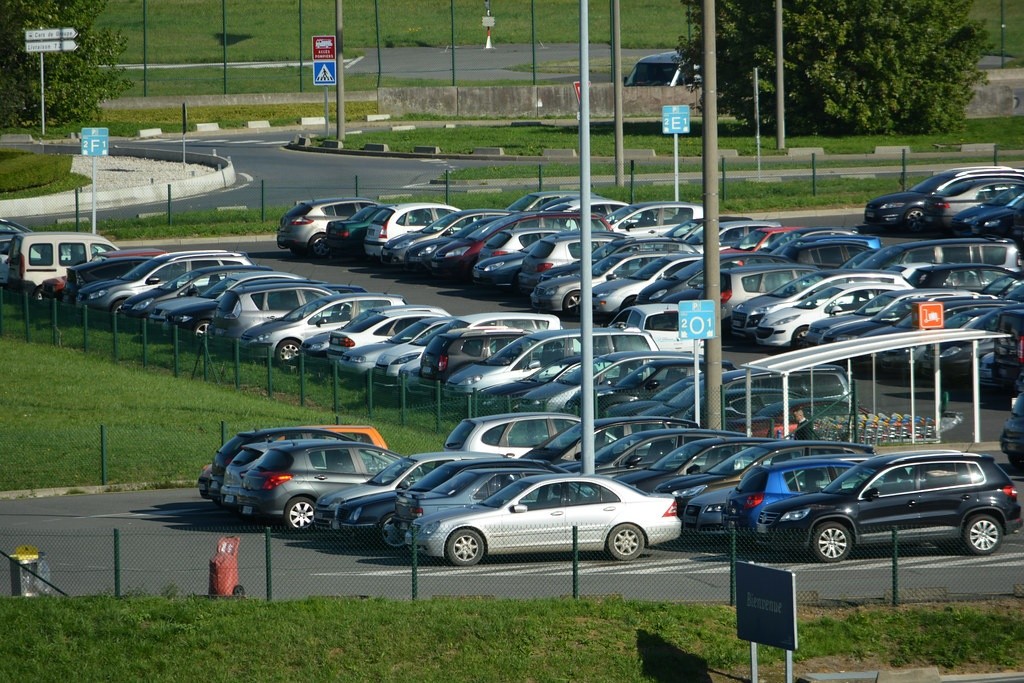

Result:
[606,303,705,356]
[275,172,1024,412]
[197,424,683,566]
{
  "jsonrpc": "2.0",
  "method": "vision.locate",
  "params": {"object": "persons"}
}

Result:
[792,409,813,440]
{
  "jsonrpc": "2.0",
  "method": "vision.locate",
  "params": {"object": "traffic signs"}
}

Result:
[25,27,79,54]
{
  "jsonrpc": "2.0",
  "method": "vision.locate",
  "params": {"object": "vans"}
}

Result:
[623,52,702,86]
[377,312,562,378]
[9,231,120,290]
[443,329,660,401]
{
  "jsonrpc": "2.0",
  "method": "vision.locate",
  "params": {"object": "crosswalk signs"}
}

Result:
[313,62,336,86]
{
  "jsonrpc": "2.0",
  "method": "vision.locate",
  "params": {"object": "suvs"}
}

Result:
[865,166,1024,234]
[1,224,852,430]
[443,411,1023,562]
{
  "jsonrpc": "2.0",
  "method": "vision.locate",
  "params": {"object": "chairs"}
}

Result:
[340,305,350,322]
[805,469,824,491]
[895,469,917,491]
[546,485,562,507]
[548,343,562,361]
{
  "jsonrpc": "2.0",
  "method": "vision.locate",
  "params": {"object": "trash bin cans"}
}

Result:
[9,554,50,596]
[209,536,246,596]
